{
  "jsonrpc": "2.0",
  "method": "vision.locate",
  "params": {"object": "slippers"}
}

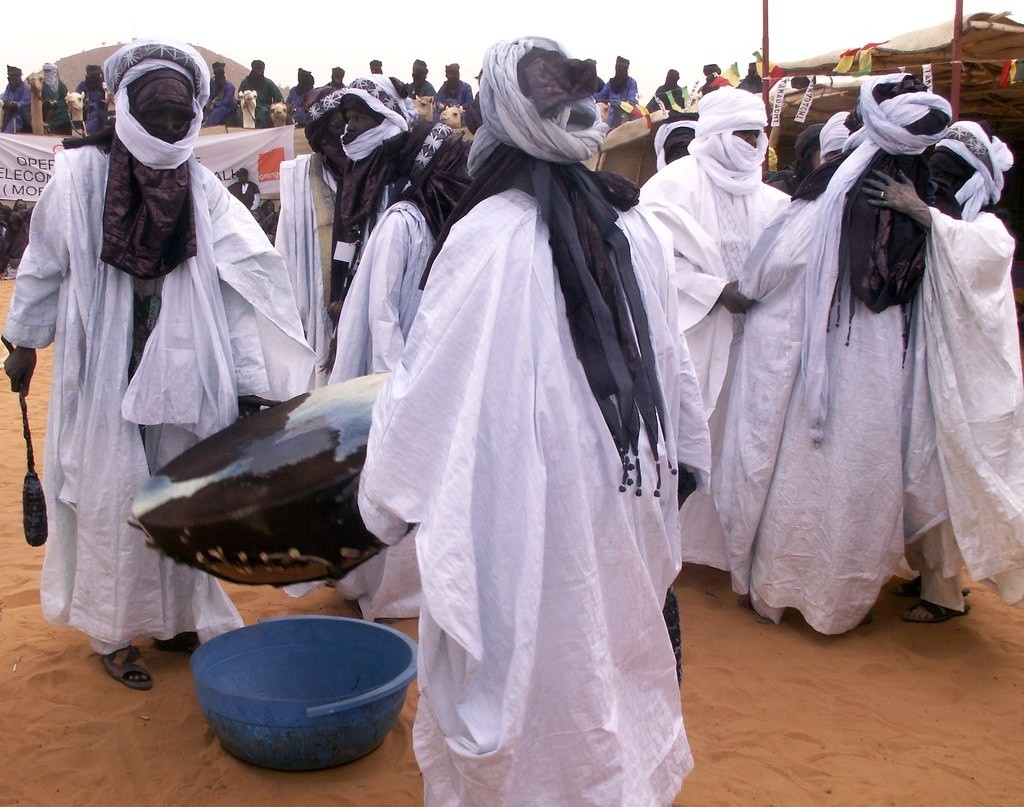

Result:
[152,629,201,652]
[860,613,872,627]
[902,600,968,622]
[101,644,153,689]
[736,593,771,624]
[890,575,923,597]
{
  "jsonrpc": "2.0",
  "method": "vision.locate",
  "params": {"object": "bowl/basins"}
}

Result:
[190,614,418,771]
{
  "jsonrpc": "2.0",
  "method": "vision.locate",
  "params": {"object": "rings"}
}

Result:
[880,191,884,198]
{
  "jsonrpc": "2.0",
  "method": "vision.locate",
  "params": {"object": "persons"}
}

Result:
[737,62,763,94]
[274,35,711,807]
[593,56,638,128]
[696,64,730,99]
[2,38,317,689]
[0,198,34,277]
[369,60,383,75]
[0,64,32,134]
[238,60,285,128]
[433,63,474,125]
[328,67,347,87]
[613,75,1024,636]
[653,69,679,112]
[286,68,315,126]
[75,64,108,134]
[204,62,237,125]
[227,168,279,243]
[410,59,436,101]
[42,62,73,136]
